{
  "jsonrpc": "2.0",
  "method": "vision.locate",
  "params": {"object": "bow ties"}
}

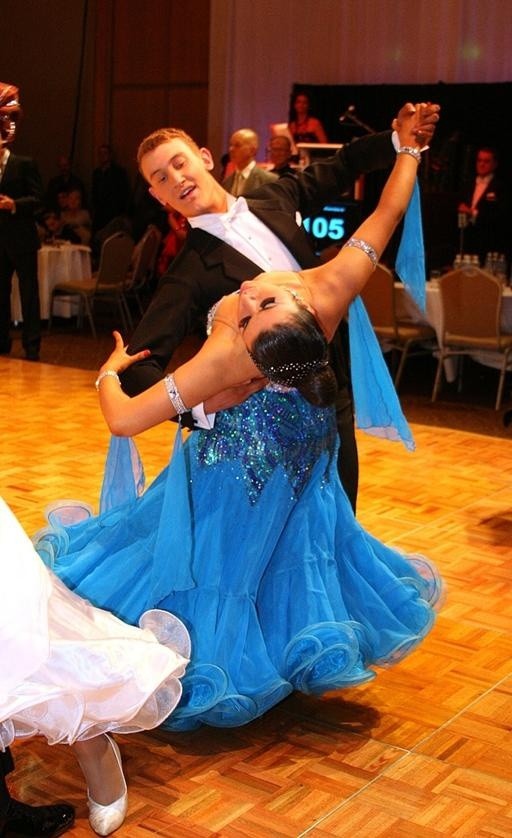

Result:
[221,195,249,229]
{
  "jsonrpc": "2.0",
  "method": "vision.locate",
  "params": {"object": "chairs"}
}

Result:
[315,243,511,412]
[7,222,163,343]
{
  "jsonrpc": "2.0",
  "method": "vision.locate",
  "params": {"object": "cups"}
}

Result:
[430,270,440,289]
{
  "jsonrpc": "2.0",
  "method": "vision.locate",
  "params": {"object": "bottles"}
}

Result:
[485,252,506,291]
[452,255,479,271]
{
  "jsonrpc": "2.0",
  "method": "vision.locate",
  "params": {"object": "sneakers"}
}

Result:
[24,348,41,361]
[1,337,13,356]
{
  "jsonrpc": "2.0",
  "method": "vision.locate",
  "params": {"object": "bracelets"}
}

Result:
[397,146,421,164]
[94,371,121,391]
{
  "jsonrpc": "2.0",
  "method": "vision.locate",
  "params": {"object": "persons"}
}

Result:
[111,98,444,530]
[0,500,192,837]
[0,148,46,363]
[35,92,441,731]
[35,88,511,393]
[0,746,74,838]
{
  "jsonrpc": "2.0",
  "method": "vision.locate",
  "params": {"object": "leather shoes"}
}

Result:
[0,798,75,837]
[86,731,128,837]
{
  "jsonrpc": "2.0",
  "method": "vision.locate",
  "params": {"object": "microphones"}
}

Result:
[339,105,355,121]
